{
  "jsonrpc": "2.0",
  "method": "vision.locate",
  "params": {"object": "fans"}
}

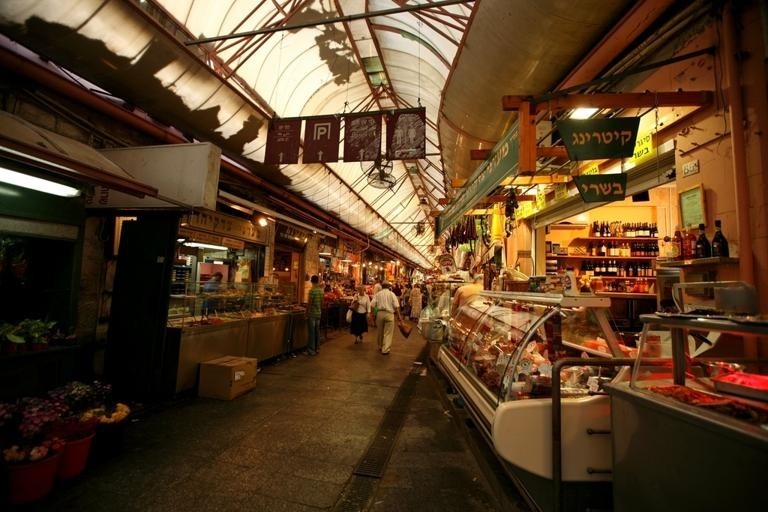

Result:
[359,148,392,178]
[366,172,395,189]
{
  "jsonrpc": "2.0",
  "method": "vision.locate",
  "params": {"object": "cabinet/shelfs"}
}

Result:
[554,238,658,297]
[164,292,289,333]
[442,290,625,411]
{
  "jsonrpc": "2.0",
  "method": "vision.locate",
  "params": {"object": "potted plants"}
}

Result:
[24,319,56,350]
[0,319,27,352]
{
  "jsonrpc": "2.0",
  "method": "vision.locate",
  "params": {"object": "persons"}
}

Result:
[326,270,507,332]
[203,272,224,314]
[304,274,325,358]
[370,281,403,355]
[345,282,373,346]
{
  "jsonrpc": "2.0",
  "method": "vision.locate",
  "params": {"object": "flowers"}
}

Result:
[0,380,133,463]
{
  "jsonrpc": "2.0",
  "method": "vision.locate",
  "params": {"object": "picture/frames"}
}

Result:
[678,183,707,231]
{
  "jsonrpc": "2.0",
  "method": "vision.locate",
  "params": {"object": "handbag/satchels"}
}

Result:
[349,300,360,310]
[345,310,353,323]
[398,324,413,338]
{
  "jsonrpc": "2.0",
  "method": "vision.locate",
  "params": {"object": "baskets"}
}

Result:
[504,280,531,291]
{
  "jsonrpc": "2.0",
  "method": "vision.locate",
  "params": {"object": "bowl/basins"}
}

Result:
[685,361,744,380]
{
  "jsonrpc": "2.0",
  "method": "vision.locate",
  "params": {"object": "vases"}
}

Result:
[0,430,96,501]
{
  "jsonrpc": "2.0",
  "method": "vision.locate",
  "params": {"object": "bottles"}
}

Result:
[563,267,578,296]
[711,220,729,257]
[580,219,659,292]
[682,223,696,258]
[671,225,683,260]
[695,223,711,257]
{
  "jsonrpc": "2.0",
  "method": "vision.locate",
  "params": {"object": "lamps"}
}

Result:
[340,253,351,262]
[415,198,428,210]
[317,241,332,256]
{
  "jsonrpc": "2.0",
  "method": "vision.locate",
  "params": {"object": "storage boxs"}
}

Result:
[197,355,257,401]
